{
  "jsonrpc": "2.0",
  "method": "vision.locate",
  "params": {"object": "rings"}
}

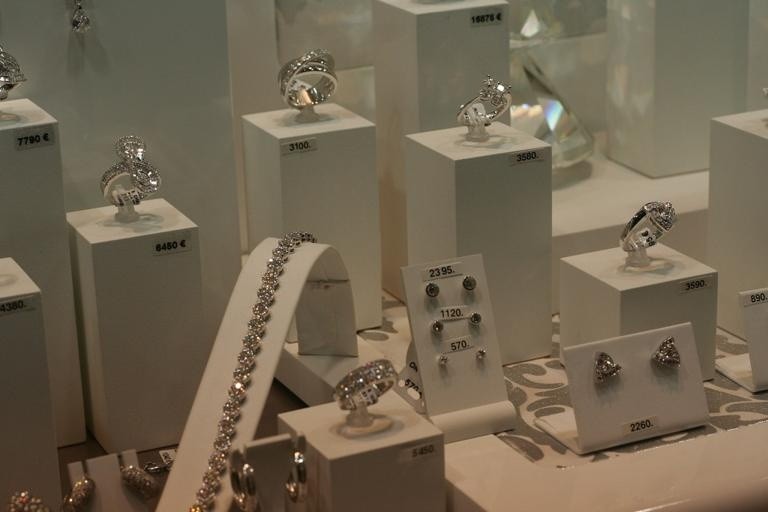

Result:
[0,46,26,100]
[455,74,513,126]
[100,134,161,208]
[277,48,338,110]
[330,359,399,411]
[72,0,91,34]
[285,449,308,504]
[228,450,258,512]
[619,200,679,252]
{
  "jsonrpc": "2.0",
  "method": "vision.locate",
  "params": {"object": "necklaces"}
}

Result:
[187,231,342,512]
